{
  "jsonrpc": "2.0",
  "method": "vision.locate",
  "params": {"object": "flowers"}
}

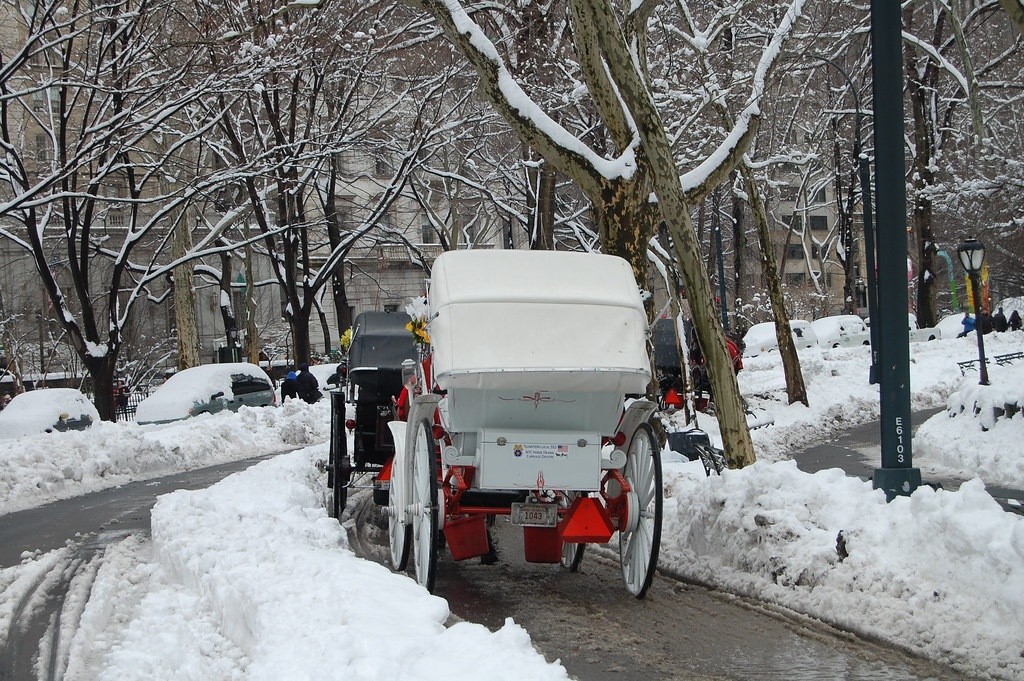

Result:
[341,329,350,351]
[405,294,430,348]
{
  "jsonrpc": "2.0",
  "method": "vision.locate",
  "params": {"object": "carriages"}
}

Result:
[655,317,746,411]
[323,247,664,596]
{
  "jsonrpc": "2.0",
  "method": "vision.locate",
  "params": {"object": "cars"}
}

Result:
[812,314,870,350]
[742,320,818,359]
[275,364,358,407]
[136,362,276,426]
[0,388,100,434]
[935,312,975,338]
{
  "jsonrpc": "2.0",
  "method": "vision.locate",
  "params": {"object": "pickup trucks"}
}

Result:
[864,313,942,345]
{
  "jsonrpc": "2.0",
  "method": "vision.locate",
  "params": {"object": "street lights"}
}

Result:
[956,236,991,385]
[858,147,880,385]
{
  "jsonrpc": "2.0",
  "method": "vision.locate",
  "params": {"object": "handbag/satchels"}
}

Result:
[306,389,323,402]
[1014,320,1022,327]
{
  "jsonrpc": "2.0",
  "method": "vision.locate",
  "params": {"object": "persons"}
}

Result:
[961,306,1022,337]
[280,356,323,405]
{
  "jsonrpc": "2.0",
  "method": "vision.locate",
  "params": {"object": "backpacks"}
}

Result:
[725,333,746,354]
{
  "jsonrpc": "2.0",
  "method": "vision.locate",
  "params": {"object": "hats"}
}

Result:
[998,308,1004,310]
[287,371,296,380]
